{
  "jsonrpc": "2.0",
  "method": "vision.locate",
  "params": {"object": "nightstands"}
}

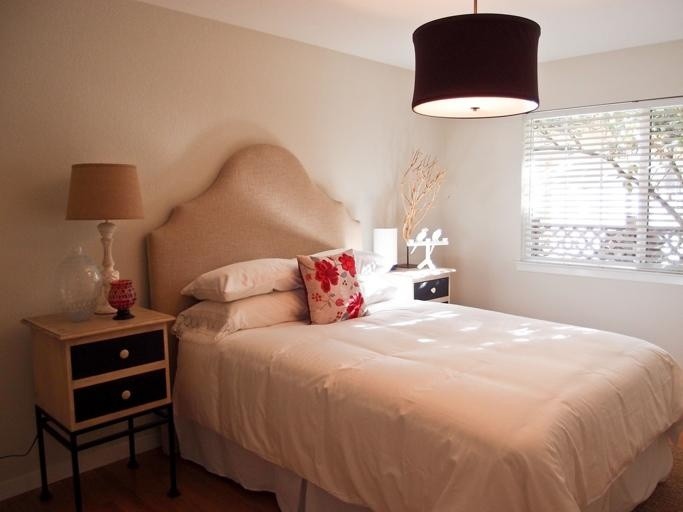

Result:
[19,304,180,512]
[398,267,456,310]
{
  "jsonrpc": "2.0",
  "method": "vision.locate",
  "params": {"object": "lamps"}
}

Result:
[412,0,542,119]
[108,280,137,320]
[64,162,147,315]
[373,228,398,272]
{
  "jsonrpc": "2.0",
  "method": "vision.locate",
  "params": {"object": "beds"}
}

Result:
[146,144,683,512]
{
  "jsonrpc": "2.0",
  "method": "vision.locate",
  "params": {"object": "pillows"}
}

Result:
[176,248,388,337]
